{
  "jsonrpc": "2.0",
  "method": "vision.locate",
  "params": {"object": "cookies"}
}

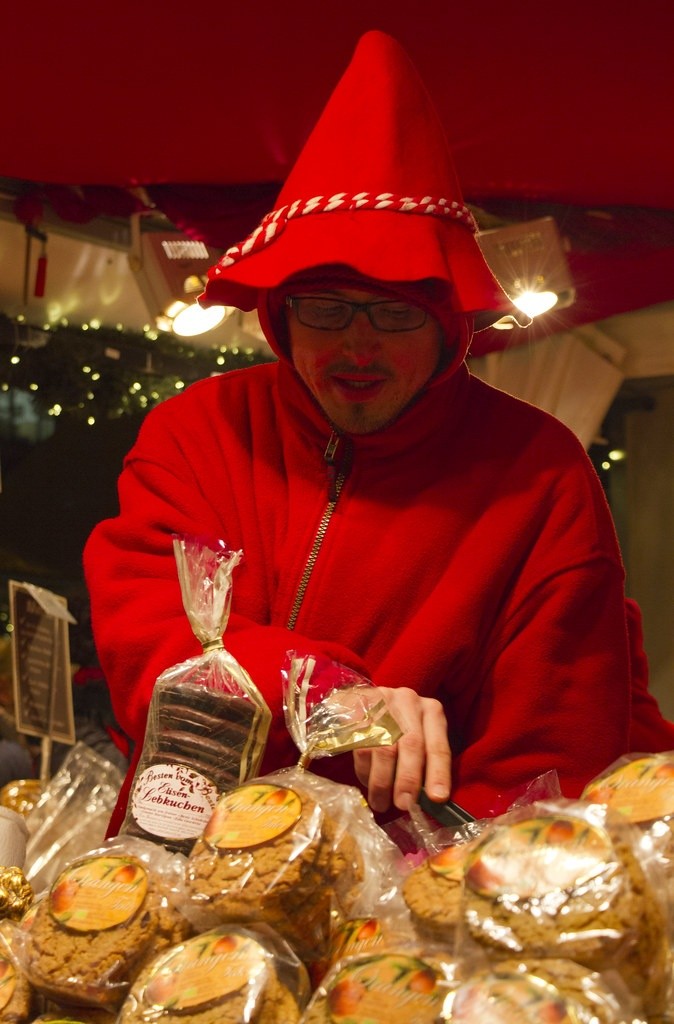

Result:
[0,683,674,1024]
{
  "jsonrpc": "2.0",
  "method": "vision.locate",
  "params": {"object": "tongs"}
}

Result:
[419,787,477,826]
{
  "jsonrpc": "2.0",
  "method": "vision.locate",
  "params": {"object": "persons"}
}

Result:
[82,29,674,867]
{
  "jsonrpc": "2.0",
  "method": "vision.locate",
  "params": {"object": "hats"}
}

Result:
[195,28,533,335]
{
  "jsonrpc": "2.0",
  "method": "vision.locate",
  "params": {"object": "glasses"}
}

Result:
[283,295,429,332]
